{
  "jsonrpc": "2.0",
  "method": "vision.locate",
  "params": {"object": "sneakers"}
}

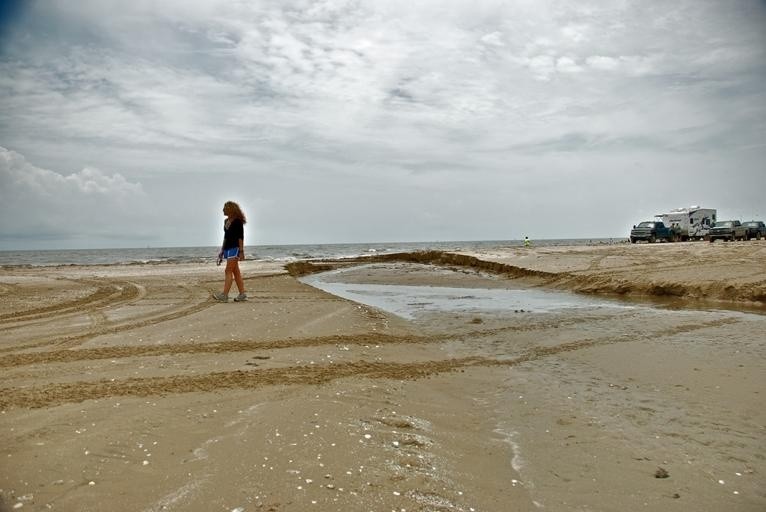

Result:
[234,294,248,301]
[213,292,228,303]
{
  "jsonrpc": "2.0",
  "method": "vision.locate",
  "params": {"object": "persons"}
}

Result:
[524,236,530,249]
[670,222,681,242]
[212,201,249,302]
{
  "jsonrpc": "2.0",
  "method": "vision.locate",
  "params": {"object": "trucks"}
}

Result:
[655,206,717,240]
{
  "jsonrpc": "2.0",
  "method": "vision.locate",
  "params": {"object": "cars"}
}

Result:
[709,220,741,242]
[735,222,766,240]
[631,221,676,243]
